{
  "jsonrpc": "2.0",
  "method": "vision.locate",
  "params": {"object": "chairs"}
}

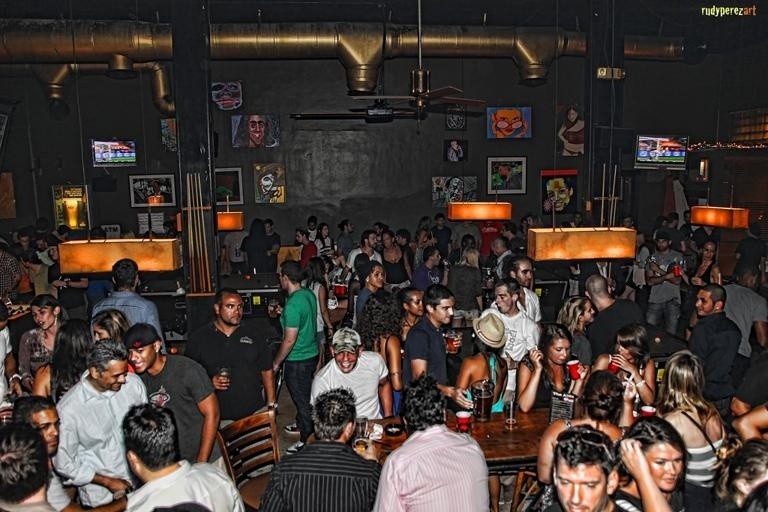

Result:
[215,407,281,509]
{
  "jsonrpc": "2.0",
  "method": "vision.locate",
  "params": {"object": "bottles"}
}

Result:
[674,264,680,277]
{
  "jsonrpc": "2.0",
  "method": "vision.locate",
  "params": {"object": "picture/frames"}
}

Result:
[51,185,92,231]
[485,106,532,140]
[214,166,245,206]
[128,173,177,209]
[486,157,527,195]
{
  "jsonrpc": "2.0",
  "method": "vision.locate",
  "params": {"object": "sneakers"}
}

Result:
[285,440,305,455]
[285,423,301,434]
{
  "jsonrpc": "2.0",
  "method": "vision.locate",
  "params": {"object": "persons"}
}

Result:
[244,114,266,149]
[557,105,585,157]
[433,176,464,208]
[447,140,463,163]
[0,211,767,512]
[133,179,169,203]
[544,176,573,215]
[489,107,525,138]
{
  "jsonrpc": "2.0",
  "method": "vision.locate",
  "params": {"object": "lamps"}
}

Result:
[527,2,637,262]
[215,196,245,231]
[448,55,513,220]
[413,97,428,136]
[690,51,749,229]
[58,72,183,273]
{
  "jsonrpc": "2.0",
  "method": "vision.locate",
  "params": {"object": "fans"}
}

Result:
[351,0,486,135]
[289,2,428,123]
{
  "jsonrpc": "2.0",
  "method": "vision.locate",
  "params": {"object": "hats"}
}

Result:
[472,313,507,348]
[332,327,362,354]
[124,322,161,349]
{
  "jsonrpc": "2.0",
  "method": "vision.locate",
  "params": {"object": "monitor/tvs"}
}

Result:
[91,137,139,169]
[633,132,689,172]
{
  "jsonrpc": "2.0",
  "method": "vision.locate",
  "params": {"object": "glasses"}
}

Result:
[557,430,614,461]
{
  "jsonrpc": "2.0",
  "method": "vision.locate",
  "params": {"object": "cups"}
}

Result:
[639,406,657,416]
[504,400,518,429]
[356,416,368,439]
[608,356,619,372]
[456,411,471,434]
[567,360,580,379]
[472,380,494,422]
[443,259,450,268]
[448,334,458,354]
[335,283,346,297]
[269,299,279,318]
[217,365,231,392]
[355,439,366,453]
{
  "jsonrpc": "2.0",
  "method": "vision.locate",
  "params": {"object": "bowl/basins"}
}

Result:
[385,423,403,436]
